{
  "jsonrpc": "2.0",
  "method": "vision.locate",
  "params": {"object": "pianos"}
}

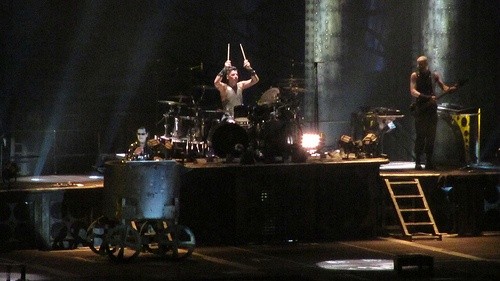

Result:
[408,78,467,118]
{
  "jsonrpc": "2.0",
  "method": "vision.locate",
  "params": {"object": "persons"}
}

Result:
[214,58,259,117]
[410,56,456,169]
[127,127,155,161]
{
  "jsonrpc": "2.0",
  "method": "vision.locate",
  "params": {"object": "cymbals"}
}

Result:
[278,78,309,93]
[193,86,218,89]
[207,108,229,113]
[170,95,189,99]
[259,88,280,105]
[157,100,185,106]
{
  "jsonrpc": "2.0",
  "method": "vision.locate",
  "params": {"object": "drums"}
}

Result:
[174,115,196,141]
[205,102,289,161]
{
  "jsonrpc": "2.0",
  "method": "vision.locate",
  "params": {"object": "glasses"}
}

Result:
[137,133,146,135]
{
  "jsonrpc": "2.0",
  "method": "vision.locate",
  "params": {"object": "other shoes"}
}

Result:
[426,164,435,169]
[415,164,422,169]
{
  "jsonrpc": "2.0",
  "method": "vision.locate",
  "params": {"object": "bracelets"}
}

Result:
[219,68,225,76]
[246,68,256,74]
